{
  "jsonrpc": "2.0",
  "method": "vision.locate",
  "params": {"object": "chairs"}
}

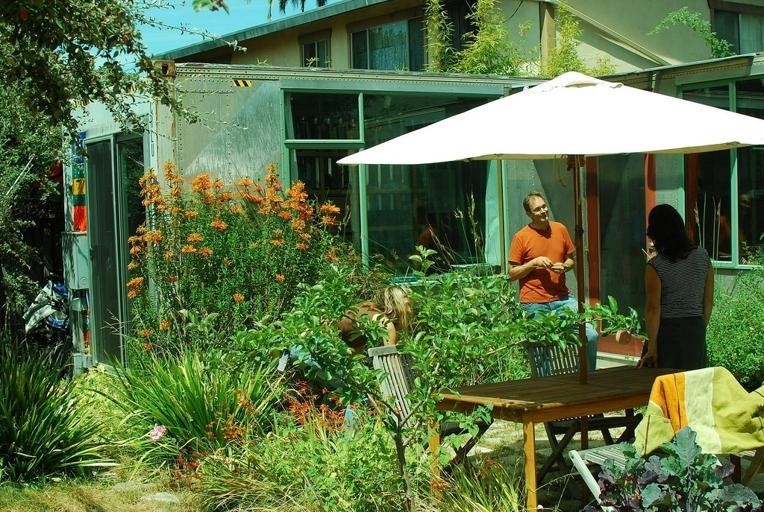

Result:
[571,364,748,511]
[526,334,642,486]
[367,344,494,487]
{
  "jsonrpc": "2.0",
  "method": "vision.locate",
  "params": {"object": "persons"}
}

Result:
[634,204,715,371]
[507,191,599,377]
[287,284,413,475]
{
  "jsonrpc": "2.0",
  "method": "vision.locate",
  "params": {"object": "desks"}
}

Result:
[424,364,689,511]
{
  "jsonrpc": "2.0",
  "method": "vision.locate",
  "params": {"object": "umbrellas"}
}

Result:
[334,69,764,451]
